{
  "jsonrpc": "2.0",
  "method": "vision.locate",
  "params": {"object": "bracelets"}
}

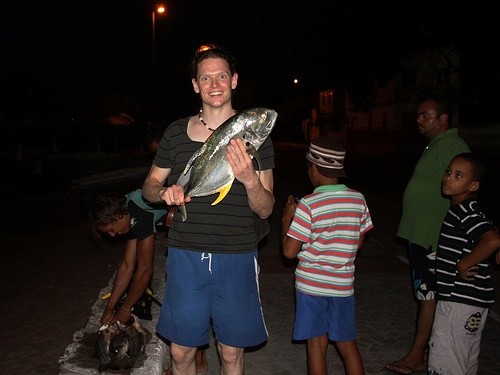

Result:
[122,304,132,313]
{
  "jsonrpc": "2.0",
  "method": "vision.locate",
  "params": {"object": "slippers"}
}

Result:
[383,360,426,375]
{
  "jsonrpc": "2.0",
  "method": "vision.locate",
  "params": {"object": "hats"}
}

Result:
[306,138,347,178]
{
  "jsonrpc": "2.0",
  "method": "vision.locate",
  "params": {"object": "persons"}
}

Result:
[383,96,473,375]
[87,188,175,324]
[280,134,374,375]
[141,49,276,375]
[426,153,500,375]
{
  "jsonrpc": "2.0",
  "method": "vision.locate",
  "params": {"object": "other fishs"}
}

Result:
[94,314,146,368]
[176,107,277,222]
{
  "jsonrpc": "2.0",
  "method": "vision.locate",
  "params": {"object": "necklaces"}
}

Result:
[198,108,215,132]
